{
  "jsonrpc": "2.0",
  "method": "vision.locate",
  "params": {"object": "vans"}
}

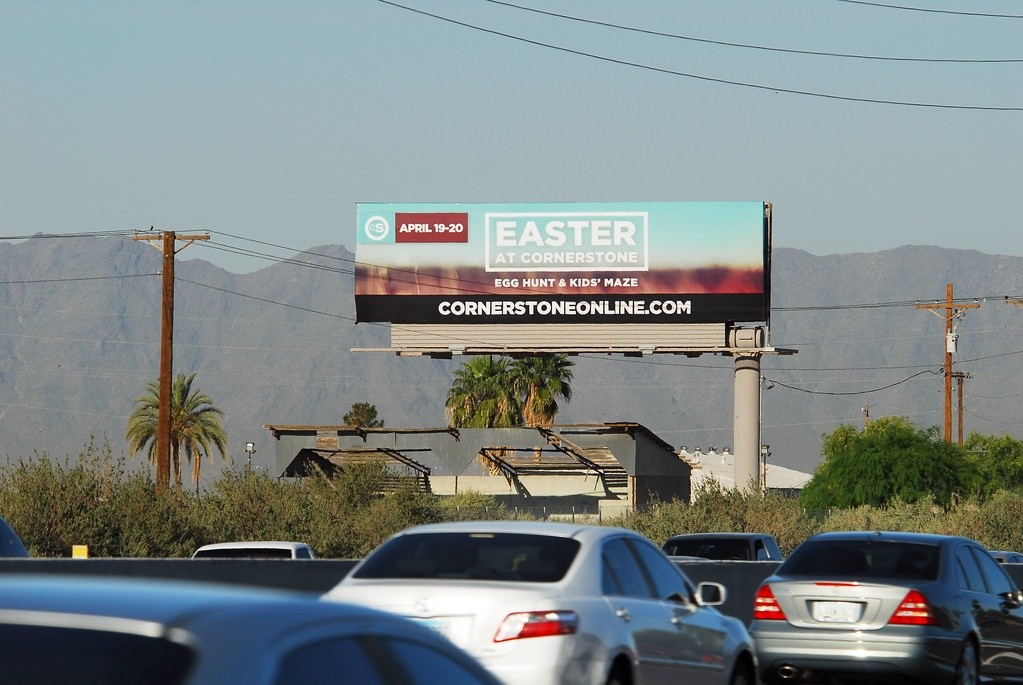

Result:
[191,540,317,560]
[660,533,786,562]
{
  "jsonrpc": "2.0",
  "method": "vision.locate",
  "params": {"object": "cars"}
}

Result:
[0,517,30,558]
[749,531,1023,685]
[318,518,762,685]
[0,571,505,685]
[986,549,1023,564]
